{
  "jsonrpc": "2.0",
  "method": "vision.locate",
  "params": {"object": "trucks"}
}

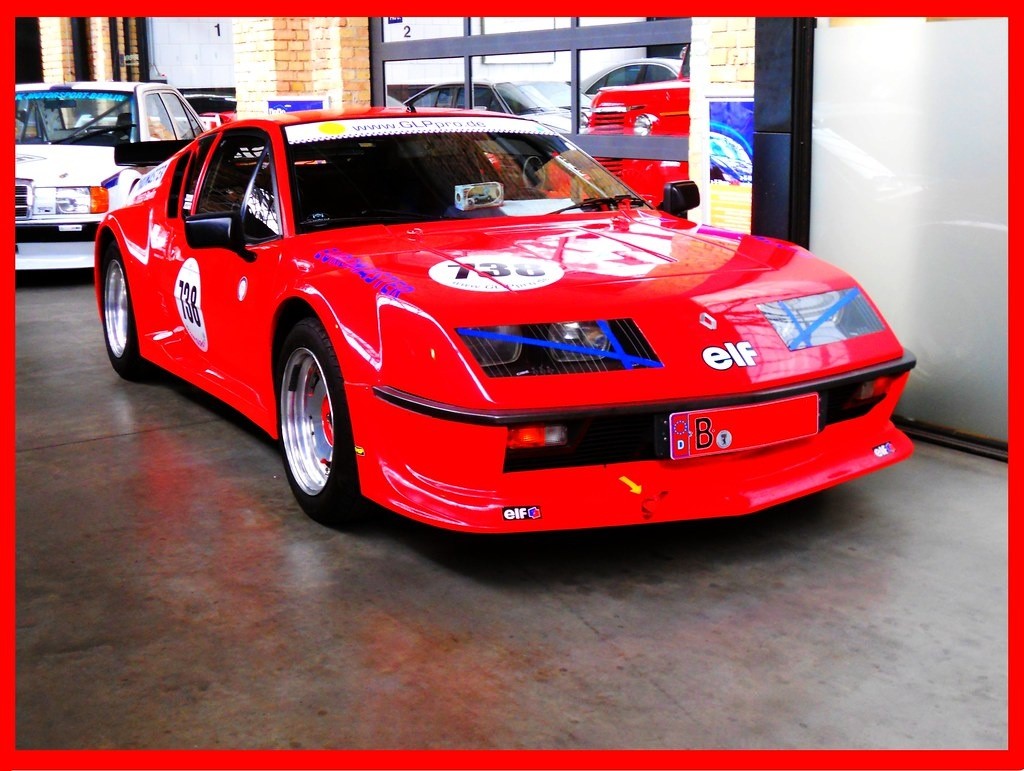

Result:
[593,40,690,214]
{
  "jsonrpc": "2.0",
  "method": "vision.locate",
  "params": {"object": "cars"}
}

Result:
[14,81,207,237]
[90,101,915,535]
[570,55,683,97]
[403,81,594,138]
